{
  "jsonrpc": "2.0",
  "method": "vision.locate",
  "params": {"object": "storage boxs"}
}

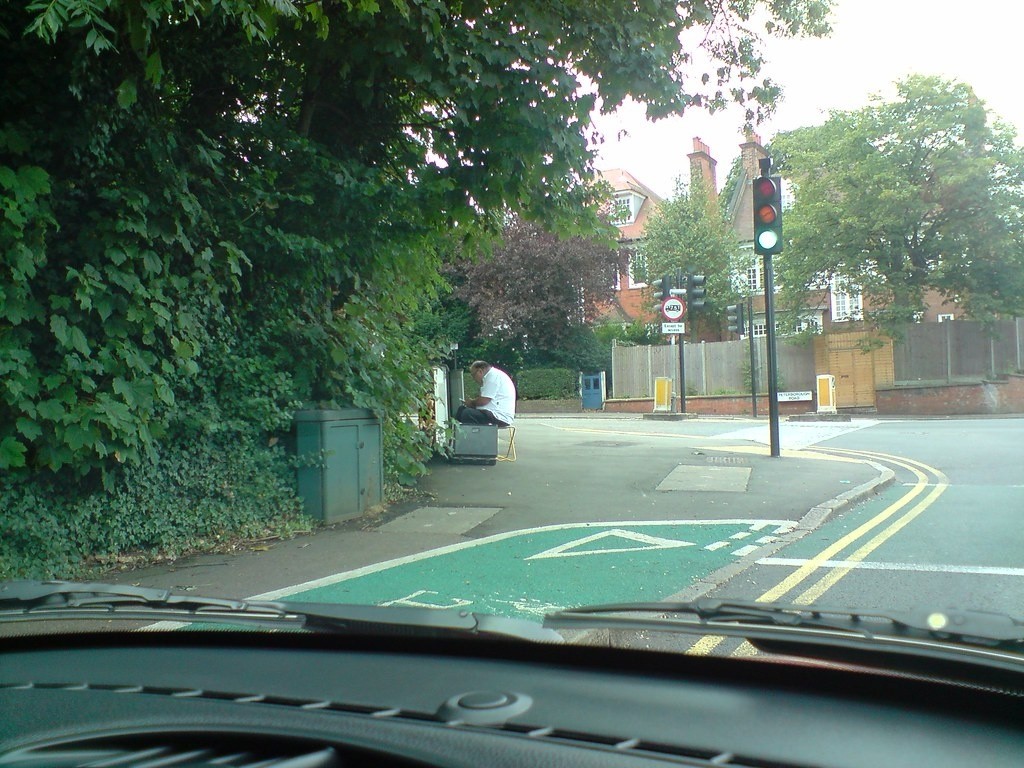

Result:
[454,422,498,456]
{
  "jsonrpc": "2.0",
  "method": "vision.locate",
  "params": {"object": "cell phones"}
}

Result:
[459,397,466,403]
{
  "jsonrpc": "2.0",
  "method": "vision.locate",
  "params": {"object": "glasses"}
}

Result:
[471,367,479,378]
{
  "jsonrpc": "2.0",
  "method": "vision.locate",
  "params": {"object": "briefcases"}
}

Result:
[448,424,498,466]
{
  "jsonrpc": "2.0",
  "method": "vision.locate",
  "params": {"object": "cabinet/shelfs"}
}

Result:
[296,406,385,526]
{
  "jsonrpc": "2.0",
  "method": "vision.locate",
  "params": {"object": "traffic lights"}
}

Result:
[751,176,785,258]
[652,274,670,313]
[686,271,707,321]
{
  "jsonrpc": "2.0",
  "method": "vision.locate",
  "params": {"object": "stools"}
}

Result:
[496,425,516,464]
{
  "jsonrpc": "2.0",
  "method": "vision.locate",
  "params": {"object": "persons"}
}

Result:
[455,361,515,428]
